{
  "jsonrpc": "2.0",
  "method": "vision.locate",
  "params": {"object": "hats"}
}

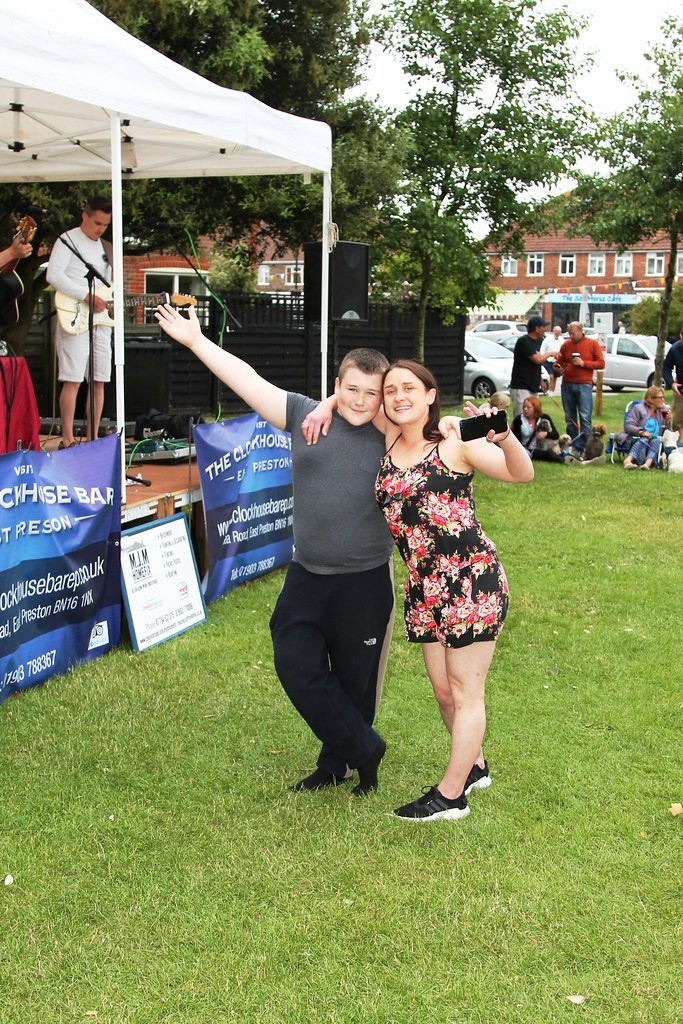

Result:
[526,316,550,332]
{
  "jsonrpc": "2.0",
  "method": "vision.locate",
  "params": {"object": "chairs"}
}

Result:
[610,400,670,470]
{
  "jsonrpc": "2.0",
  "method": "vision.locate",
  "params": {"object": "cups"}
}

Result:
[572,352,581,365]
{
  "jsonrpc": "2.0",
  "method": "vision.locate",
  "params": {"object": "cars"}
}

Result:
[465,320,528,342]
[561,327,596,339]
[496,334,525,353]
[464,336,550,399]
[592,334,676,391]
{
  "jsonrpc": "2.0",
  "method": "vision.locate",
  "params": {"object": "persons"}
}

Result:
[509,315,562,431]
[154,298,394,798]
[46,196,114,447]
[613,320,631,334]
[0,232,33,269]
[617,386,672,471]
[553,321,605,456]
[301,359,533,824]
[540,326,566,398]
[661,330,683,447]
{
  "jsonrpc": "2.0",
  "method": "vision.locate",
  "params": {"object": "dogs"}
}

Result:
[659,429,683,475]
[559,433,581,464]
[536,419,561,454]
[583,424,607,463]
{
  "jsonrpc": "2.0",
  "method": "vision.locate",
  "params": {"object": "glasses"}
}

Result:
[651,396,665,400]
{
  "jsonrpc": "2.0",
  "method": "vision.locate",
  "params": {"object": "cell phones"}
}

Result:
[460,409,508,442]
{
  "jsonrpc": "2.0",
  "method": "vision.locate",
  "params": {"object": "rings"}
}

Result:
[486,435,492,441]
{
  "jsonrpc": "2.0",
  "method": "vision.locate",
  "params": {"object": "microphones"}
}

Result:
[23,204,48,214]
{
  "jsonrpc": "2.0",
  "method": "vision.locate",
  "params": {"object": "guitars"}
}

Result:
[54,282,197,333]
[0,214,38,331]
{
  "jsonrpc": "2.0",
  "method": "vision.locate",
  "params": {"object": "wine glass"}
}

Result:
[661,405,671,428]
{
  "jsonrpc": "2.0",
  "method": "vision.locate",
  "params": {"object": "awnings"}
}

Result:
[465,292,542,321]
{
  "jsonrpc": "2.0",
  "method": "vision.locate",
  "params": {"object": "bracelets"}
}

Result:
[500,429,510,441]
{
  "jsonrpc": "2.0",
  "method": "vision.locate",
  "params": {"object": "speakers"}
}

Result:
[304,241,370,322]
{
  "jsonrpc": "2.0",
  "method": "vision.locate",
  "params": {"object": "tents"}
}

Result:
[0,0,330,506]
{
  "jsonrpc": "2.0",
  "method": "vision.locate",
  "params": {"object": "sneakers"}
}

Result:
[391,784,470,823]
[464,760,492,795]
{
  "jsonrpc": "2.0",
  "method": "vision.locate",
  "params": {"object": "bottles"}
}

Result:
[561,447,571,466]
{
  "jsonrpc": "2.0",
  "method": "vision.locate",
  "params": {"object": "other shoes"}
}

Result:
[289,768,354,792]
[640,464,649,471]
[58,441,78,450]
[624,463,637,470]
[548,391,555,397]
[351,739,386,797]
